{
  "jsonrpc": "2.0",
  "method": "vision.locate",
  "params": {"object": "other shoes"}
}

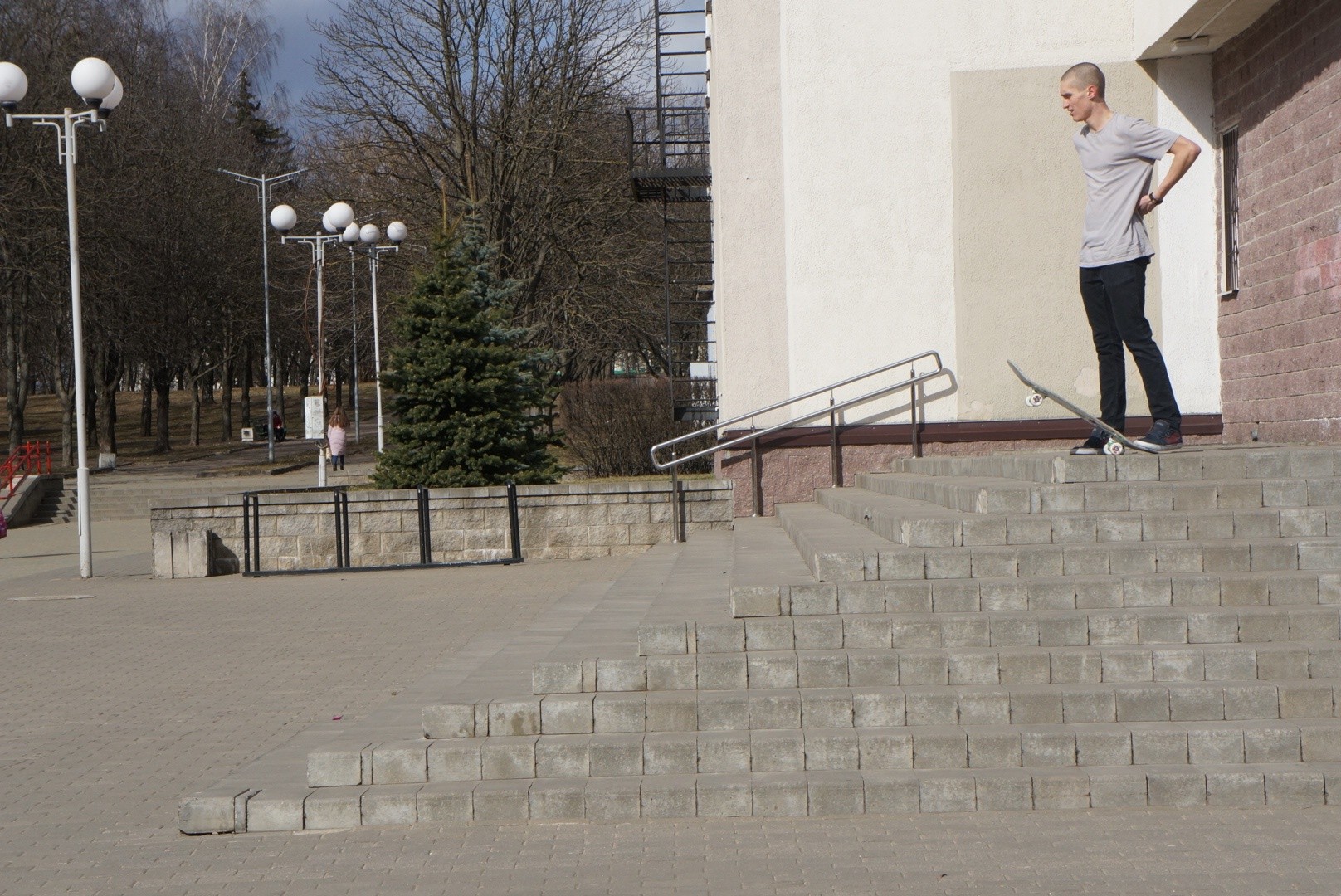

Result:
[340,466,343,470]
[333,465,337,470]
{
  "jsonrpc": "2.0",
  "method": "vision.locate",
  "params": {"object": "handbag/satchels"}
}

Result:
[325,447,332,460]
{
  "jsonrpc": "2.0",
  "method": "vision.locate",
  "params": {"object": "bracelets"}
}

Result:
[1149,193,1162,205]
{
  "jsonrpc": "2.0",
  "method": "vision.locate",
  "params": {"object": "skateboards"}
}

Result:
[1007,358,1161,455]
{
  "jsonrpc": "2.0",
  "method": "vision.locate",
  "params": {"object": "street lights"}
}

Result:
[0,57,124,580]
[269,201,407,489]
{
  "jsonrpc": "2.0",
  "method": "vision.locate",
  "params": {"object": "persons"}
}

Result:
[1059,62,1201,455]
[273,411,282,442]
[327,406,350,471]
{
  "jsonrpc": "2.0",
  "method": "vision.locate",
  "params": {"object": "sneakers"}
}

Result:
[1069,430,1125,454]
[1133,420,1183,450]
